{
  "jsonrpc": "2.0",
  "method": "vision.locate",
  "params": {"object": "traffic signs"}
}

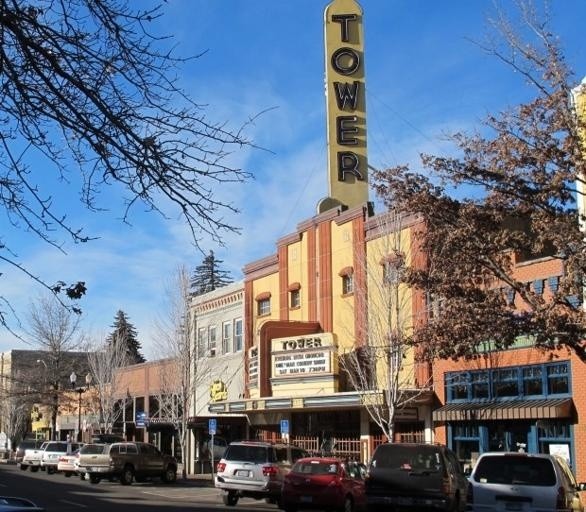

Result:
[208,420,217,436]
[280,421,287,435]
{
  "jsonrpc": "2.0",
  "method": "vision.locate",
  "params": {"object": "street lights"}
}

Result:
[70,371,92,442]
[36,359,47,391]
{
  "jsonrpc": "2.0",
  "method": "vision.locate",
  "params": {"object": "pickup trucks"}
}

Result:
[78,440,180,486]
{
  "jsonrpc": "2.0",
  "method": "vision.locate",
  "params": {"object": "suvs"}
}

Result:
[212,439,309,506]
[466,452,586,511]
[364,441,468,512]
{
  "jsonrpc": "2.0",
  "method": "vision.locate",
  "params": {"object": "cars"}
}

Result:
[282,458,367,512]
[11,439,83,480]
